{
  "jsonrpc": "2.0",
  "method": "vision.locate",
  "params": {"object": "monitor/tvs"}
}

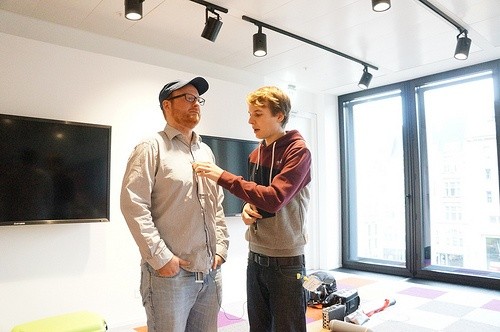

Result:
[199,135,261,216]
[0,114,112,226]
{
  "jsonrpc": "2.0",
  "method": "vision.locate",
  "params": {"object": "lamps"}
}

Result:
[242,14,379,91]
[124,0,228,43]
[372,0,472,61]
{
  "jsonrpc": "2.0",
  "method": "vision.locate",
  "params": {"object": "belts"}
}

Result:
[249,252,304,267]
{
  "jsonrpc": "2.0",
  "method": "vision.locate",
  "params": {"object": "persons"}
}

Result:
[118,76,231,332]
[191,86,312,332]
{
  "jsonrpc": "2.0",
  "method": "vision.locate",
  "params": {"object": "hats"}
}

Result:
[159,76,209,108]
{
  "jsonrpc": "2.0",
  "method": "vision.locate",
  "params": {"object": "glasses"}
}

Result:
[168,92,205,106]
